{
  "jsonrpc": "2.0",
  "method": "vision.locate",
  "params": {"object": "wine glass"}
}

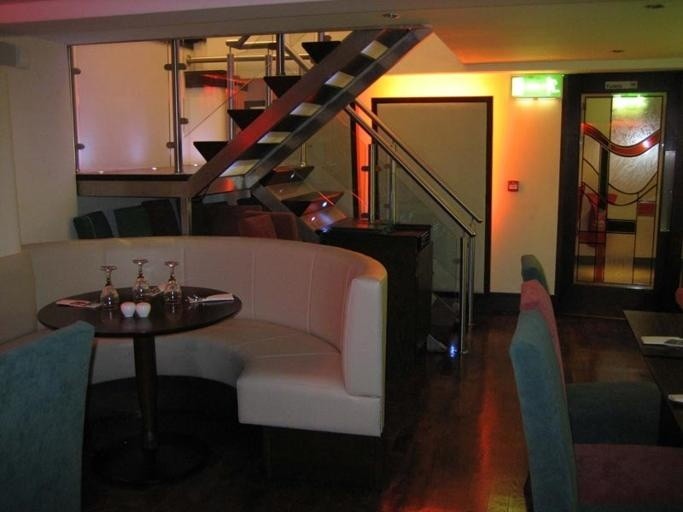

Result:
[163,261,183,305]
[97,265,120,311]
[132,259,153,304]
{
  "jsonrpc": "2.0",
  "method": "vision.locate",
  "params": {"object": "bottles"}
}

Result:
[120,301,136,318]
[135,302,151,318]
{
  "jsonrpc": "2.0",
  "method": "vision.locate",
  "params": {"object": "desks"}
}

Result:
[322,218,432,370]
[36,285,242,488]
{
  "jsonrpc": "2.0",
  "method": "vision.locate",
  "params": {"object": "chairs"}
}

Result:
[509,254,683,512]
[0,320,95,512]
[73,197,299,240]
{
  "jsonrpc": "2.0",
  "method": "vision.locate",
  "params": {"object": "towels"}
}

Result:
[199,294,233,302]
[57,299,101,308]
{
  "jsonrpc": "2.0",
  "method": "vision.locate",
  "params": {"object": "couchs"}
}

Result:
[0,236,387,486]
